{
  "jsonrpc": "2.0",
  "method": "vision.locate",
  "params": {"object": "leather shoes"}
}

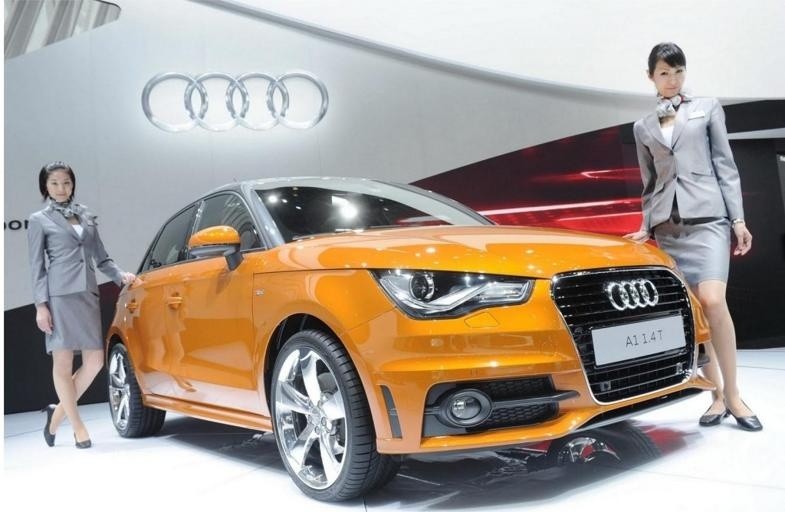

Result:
[74,432,91,449]
[723,396,763,431]
[699,398,730,426]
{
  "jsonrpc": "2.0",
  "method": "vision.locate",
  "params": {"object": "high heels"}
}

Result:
[41,403,56,447]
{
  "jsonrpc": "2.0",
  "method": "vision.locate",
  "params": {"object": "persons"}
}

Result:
[25,160,137,449]
[623,42,764,431]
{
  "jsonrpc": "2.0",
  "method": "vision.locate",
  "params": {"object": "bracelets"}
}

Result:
[731,219,745,228]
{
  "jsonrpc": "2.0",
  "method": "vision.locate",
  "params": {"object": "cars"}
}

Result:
[103,176,717,501]
